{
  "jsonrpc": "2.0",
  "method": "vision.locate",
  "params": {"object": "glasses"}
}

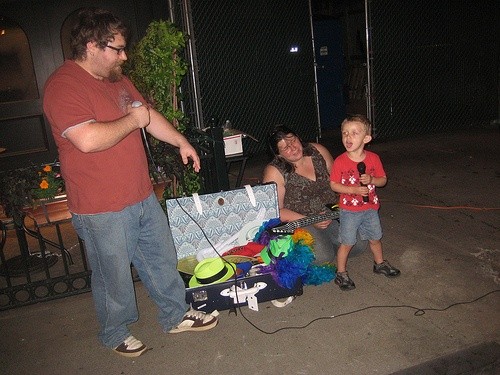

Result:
[106,44,127,56]
[282,137,298,152]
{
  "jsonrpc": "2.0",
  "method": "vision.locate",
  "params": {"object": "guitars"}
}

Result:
[270,203,340,236]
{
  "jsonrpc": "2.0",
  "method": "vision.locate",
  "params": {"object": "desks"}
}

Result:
[0,175,174,278]
[225,151,253,187]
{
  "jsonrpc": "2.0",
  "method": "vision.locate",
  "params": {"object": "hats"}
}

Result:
[189,255,237,288]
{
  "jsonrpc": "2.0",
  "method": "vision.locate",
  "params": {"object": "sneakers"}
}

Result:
[334,270,356,292]
[166,302,219,334]
[373,259,401,279]
[102,334,147,357]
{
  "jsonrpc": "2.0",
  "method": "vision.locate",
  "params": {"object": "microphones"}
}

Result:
[357,162,369,202]
[132,101,150,153]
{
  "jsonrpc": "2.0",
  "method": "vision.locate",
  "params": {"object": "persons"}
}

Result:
[329,115,401,292]
[44,8,218,359]
[263,124,340,267]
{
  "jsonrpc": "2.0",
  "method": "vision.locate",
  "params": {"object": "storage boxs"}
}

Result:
[222,130,258,156]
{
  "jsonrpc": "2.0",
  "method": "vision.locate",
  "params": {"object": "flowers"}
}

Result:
[29,161,63,203]
